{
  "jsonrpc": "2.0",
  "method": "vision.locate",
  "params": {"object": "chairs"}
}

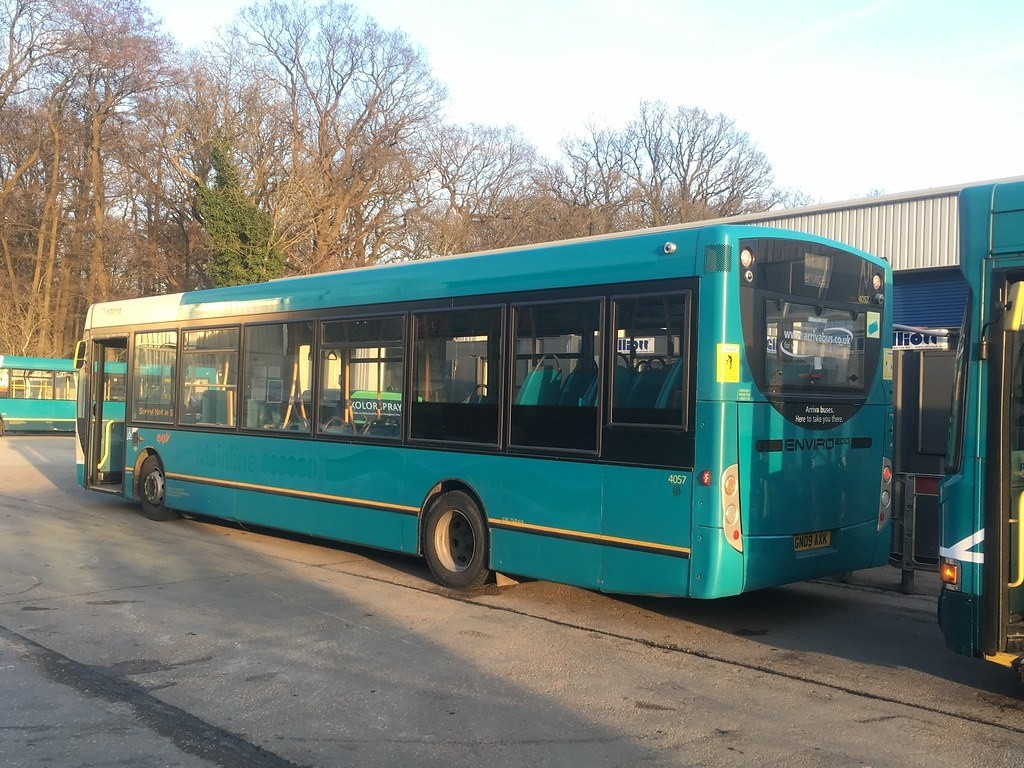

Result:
[203,352,839,456]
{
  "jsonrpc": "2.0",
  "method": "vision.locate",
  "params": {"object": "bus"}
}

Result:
[0,355,217,437]
[936,180,1024,678]
[73,224,894,600]
